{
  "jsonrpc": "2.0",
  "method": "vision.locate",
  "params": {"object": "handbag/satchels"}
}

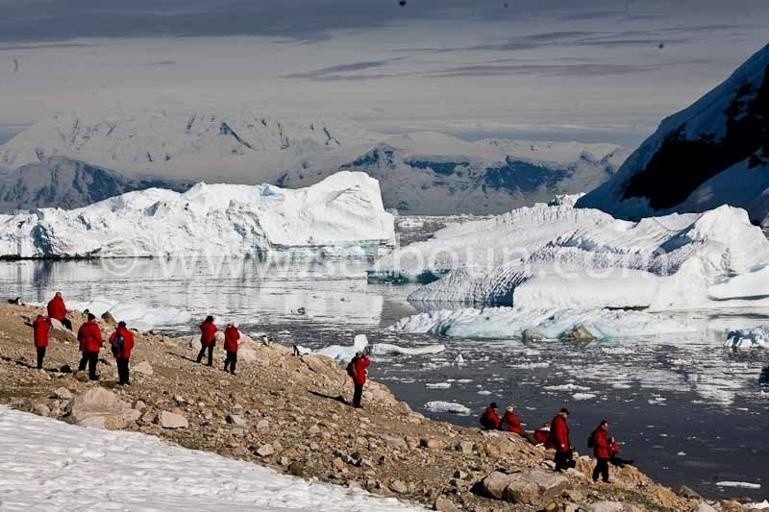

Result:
[111,335,124,352]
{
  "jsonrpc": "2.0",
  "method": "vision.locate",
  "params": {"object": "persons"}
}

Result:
[47,292,72,330]
[77,313,104,380]
[109,321,134,385]
[485,403,634,482]
[352,351,370,408]
[197,316,223,366]
[33,314,51,369]
[224,324,240,375]
